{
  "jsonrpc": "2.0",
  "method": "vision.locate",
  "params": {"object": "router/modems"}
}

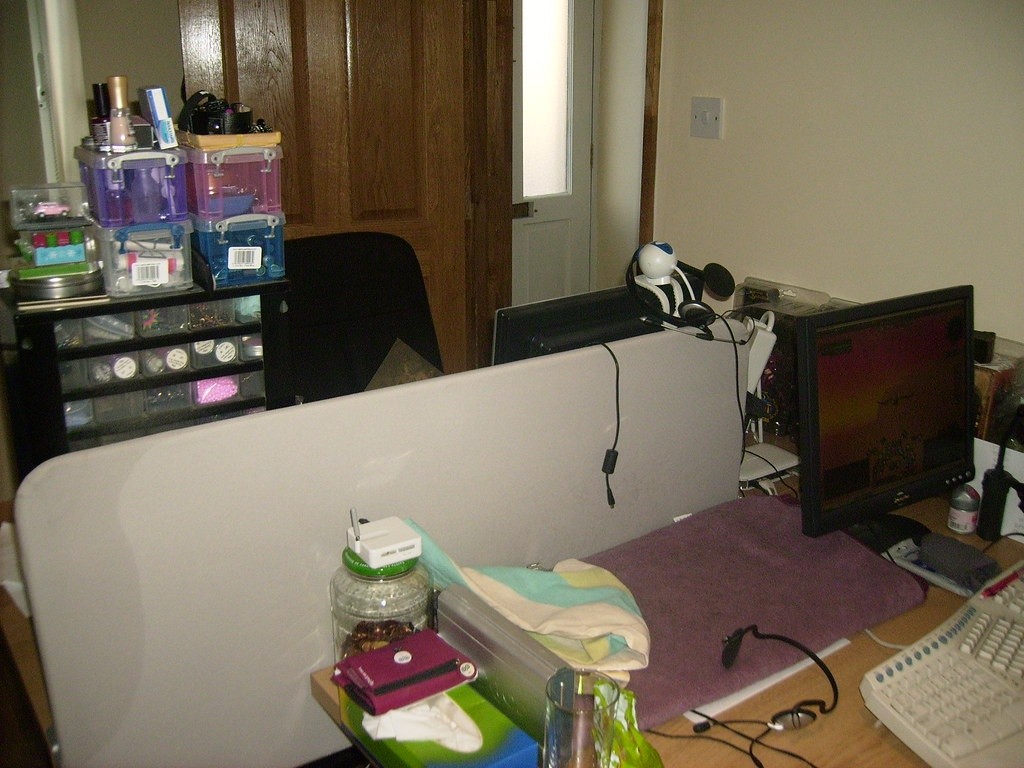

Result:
[738,378,800,490]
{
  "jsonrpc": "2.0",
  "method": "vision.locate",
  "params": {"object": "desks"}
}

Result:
[0,420,1024,768]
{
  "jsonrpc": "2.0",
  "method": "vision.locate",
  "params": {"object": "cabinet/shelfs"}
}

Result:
[0,277,289,491]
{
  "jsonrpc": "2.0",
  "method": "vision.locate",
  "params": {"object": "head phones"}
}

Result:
[626,244,734,327]
[722,624,838,730]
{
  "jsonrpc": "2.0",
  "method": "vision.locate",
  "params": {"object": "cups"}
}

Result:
[541,669,620,768]
[947,485,980,535]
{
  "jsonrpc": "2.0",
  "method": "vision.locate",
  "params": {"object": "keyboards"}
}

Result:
[857,556,1024,768]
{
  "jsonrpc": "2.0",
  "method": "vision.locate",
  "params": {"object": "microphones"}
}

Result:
[693,720,771,733]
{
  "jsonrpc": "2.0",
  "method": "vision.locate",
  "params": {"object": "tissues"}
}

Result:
[338,683,539,768]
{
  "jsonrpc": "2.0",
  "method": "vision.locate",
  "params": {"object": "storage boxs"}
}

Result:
[74,145,188,227]
[189,211,286,287]
[80,202,195,298]
[173,145,283,220]
[8,182,93,231]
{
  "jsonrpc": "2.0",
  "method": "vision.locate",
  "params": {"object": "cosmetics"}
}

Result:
[98,166,185,223]
[93,333,239,380]
[211,234,285,278]
[83,74,136,149]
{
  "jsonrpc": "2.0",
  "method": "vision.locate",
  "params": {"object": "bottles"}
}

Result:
[331,548,433,665]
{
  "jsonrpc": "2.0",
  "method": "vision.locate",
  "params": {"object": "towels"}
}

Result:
[404,495,927,733]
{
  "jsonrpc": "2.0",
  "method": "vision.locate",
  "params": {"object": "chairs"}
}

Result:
[262,231,444,412]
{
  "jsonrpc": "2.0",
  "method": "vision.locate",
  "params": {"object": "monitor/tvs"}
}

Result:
[491,273,704,366]
[796,282,976,552]
